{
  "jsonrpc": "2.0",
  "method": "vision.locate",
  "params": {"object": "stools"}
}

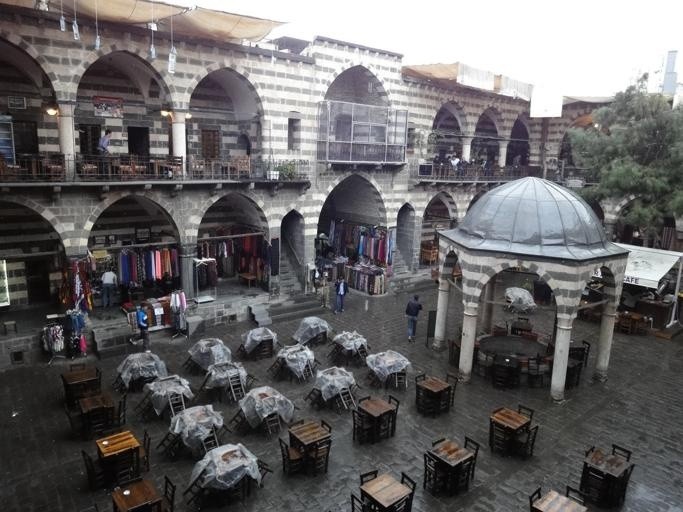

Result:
[3,321,17,335]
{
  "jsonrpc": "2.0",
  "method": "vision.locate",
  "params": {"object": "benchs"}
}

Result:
[239,273,257,289]
[91,324,136,360]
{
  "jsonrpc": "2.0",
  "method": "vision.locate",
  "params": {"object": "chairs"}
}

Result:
[75,151,183,179]
[198,362,301,438]
[182,338,232,373]
[488,404,540,457]
[422,436,480,496]
[187,151,251,180]
[278,418,334,477]
[447,318,592,392]
[236,327,280,361]
[350,469,417,511]
[60,363,127,435]
[113,351,169,395]
[267,343,323,386]
[327,329,371,368]
[182,442,273,511]
[350,394,401,446]
[579,444,636,511]
[291,318,334,348]
[528,485,590,512]
[364,349,411,390]
[80,428,177,511]
[134,374,232,455]
[0,151,65,182]
[303,366,363,414]
[414,372,460,418]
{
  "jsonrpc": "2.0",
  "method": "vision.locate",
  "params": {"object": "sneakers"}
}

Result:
[129,339,151,353]
[409,336,415,341]
[321,304,344,314]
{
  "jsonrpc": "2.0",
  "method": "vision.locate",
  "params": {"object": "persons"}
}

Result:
[334,275,349,315]
[513,155,521,166]
[100,267,118,309]
[130,303,150,353]
[433,155,494,177]
[405,295,422,342]
[97,129,112,177]
